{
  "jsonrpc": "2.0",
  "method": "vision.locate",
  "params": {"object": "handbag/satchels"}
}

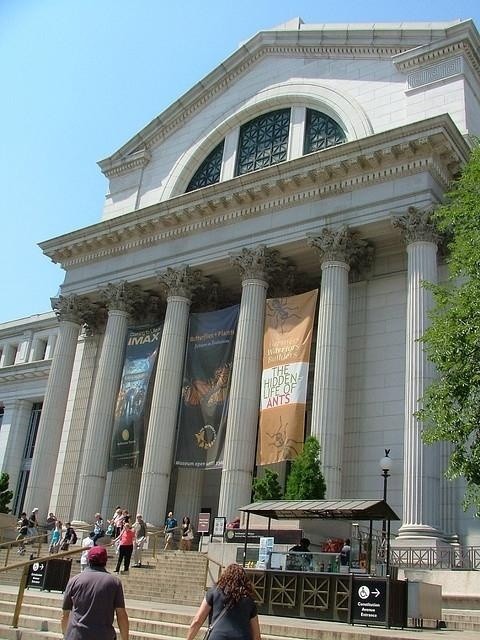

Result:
[188,532,194,539]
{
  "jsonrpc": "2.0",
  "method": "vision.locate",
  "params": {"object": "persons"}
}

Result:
[15,506,194,573]
[281,538,313,571]
[60,546,131,639]
[341,538,350,565]
[185,563,265,639]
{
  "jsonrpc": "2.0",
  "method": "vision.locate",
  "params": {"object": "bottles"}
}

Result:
[247,561,255,568]
[300,560,339,572]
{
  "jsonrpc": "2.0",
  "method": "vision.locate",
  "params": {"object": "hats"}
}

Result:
[31,508,39,513]
[88,546,108,564]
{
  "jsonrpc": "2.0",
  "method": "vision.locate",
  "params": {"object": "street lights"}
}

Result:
[380,449,394,557]
